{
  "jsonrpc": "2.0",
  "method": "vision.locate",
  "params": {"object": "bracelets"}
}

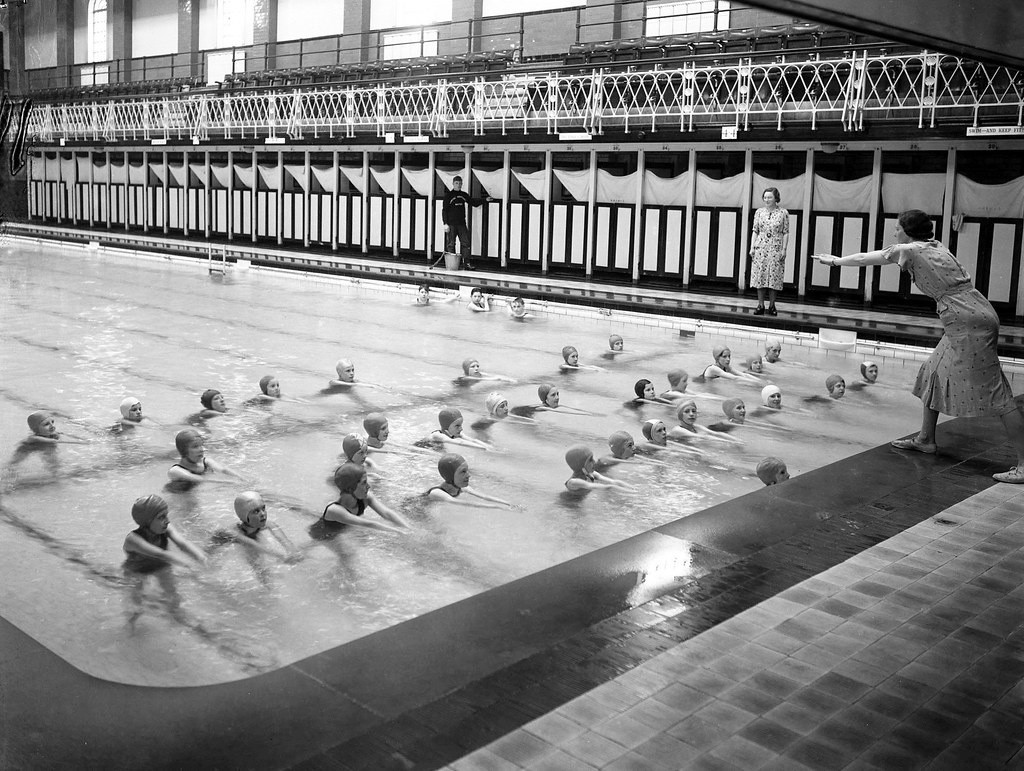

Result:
[832,258,836,266]
[783,248,786,250]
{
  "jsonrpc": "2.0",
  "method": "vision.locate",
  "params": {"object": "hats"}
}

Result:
[765,338,781,352]
[747,353,762,369]
[175,429,201,454]
[667,368,689,386]
[132,494,167,526]
[722,398,743,418]
[201,389,221,410]
[27,409,53,430]
[609,334,624,349]
[826,374,844,393]
[538,383,557,403]
[120,396,140,419]
[462,358,479,375]
[565,446,593,473]
[563,346,577,362]
[643,419,662,441]
[861,362,878,379]
[337,358,354,375]
[712,344,731,359]
[437,453,466,482]
[609,431,631,458]
[363,412,389,438]
[634,379,652,396]
[486,393,508,414]
[761,384,781,407]
[343,433,367,460]
[677,399,697,420]
[334,463,365,491]
[234,492,265,524]
[756,456,787,485]
[439,406,462,431]
[260,376,275,393]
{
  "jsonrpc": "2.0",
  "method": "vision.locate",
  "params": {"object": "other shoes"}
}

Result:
[464,263,476,270]
[891,439,936,454]
[754,304,765,316]
[769,306,778,316]
[992,466,1024,484]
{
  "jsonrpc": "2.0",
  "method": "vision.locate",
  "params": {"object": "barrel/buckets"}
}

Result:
[444,253,461,271]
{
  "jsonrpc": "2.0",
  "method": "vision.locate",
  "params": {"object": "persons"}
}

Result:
[811,209,1024,482]
[748,187,789,316]
[442,175,493,271]
[24,287,880,574]
